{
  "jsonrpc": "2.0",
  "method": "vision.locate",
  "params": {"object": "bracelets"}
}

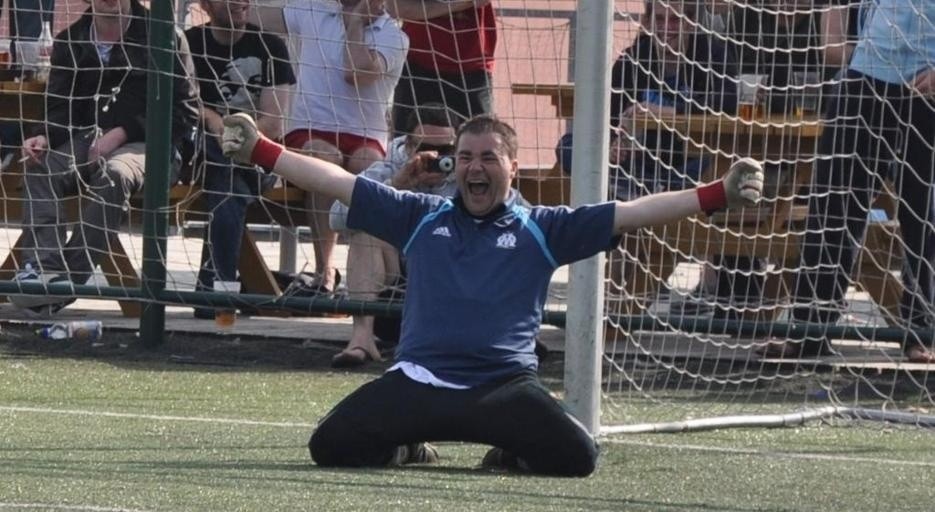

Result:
[698,181,728,217]
[248,135,284,176]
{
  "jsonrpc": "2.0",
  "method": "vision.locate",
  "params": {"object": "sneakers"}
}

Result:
[483,448,502,466]
[409,442,439,463]
[7,264,79,318]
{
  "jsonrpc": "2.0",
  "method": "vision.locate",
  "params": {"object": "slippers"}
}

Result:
[331,346,374,367]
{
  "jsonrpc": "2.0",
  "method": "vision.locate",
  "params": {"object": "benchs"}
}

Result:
[0,68,847,329]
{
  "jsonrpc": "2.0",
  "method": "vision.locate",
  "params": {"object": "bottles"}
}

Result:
[38,320,104,344]
[33,22,54,84]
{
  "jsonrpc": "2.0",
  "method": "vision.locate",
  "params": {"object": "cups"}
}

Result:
[211,281,241,328]
[736,74,769,126]
[0,39,12,76]
[791,71,820,123]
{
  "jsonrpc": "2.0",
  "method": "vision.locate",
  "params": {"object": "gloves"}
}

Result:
[221,112,285,174]
[697,157,766,216]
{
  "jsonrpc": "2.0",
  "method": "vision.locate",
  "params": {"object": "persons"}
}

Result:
[555,0,935,361]
[221,112,765,477]
[2,1,495,363]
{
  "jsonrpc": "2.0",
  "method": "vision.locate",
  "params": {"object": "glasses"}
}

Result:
[413,142,455,157]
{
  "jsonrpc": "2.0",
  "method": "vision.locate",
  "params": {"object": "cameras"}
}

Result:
[427,156,456,174]
